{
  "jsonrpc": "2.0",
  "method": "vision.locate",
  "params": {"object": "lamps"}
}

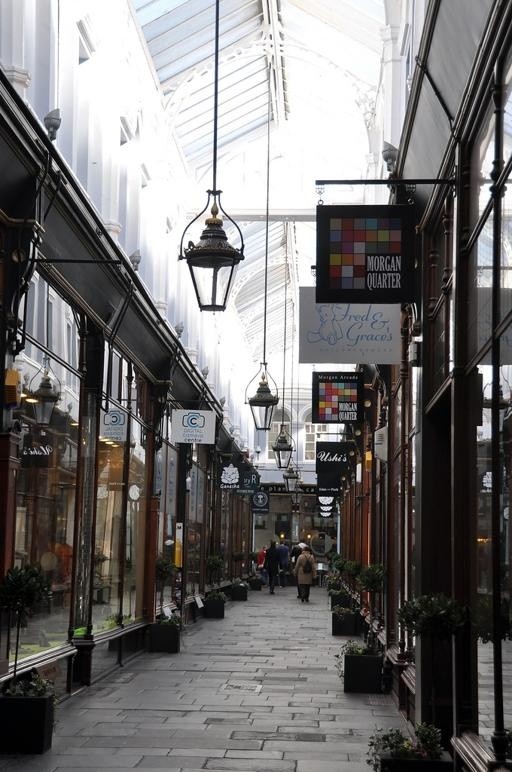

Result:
[271,220,302,509]
[28,2,62,426]
[178,0,243,313]
[245,60,279,432]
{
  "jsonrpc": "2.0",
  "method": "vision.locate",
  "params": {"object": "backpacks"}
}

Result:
[302,554,312,573]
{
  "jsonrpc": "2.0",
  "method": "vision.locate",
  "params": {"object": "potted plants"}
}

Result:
[344,560,386,693]
[365,592,455,772]
[203,552,263,618]
[150,554,181,653]
[322,551,362,636]
[0,561,54,756]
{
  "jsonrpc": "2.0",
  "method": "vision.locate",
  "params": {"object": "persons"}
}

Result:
[278,540,289,590]
[290,537,309,598]
[293,547,317,603]
[257,540,280,594]
